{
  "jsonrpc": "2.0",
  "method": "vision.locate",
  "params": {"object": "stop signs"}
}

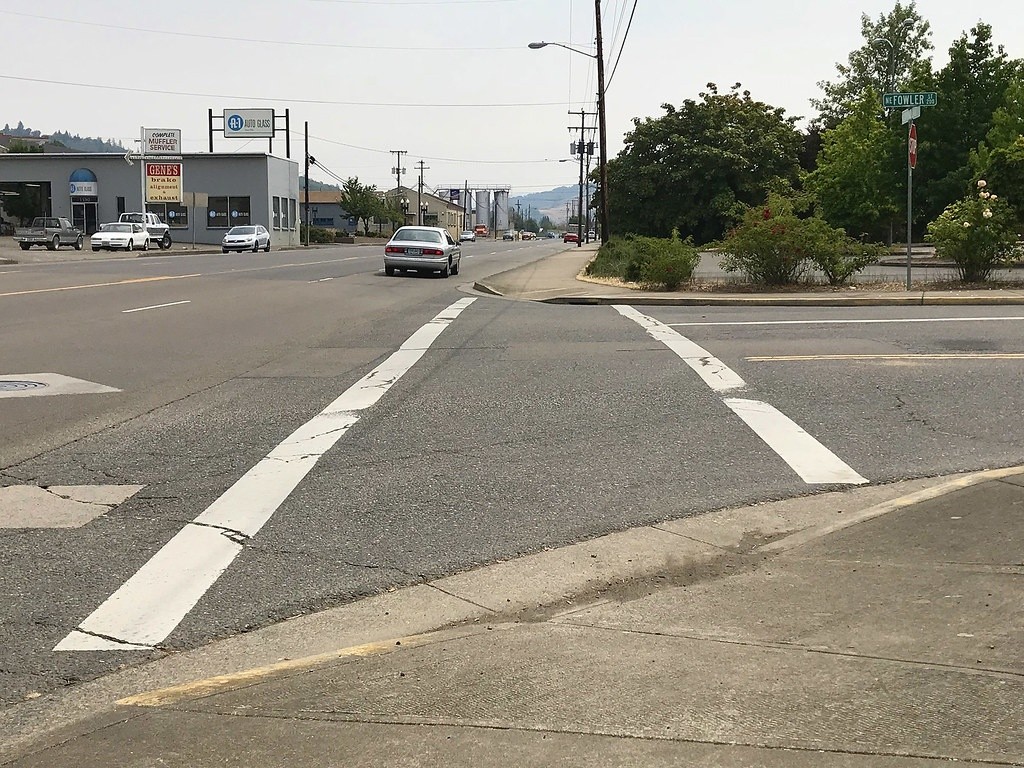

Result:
[908,122,919,170]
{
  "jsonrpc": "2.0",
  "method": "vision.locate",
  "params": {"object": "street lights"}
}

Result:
[527,41,611,246]
[375,193,386,232]
[419,201,428,226]
[871,17,914,245]
[558,158,590,243]
[400,199,410,226]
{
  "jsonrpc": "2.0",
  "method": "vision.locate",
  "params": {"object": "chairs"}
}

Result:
[47,220,52,226]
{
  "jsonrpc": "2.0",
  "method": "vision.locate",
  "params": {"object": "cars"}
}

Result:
[221,224,271,254]
[502,230,513,240]
[90,222,151,252]
[382,224,461,279]
[459,230,476,242]
[559,224,595,244]
[547,232,555,238]
[521,231,536,240]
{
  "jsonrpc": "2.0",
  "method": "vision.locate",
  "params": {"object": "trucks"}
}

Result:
[474,223,489,237]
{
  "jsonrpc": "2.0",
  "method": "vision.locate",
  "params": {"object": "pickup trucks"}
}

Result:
[12,217,84,251]
[98,211,173,250]
